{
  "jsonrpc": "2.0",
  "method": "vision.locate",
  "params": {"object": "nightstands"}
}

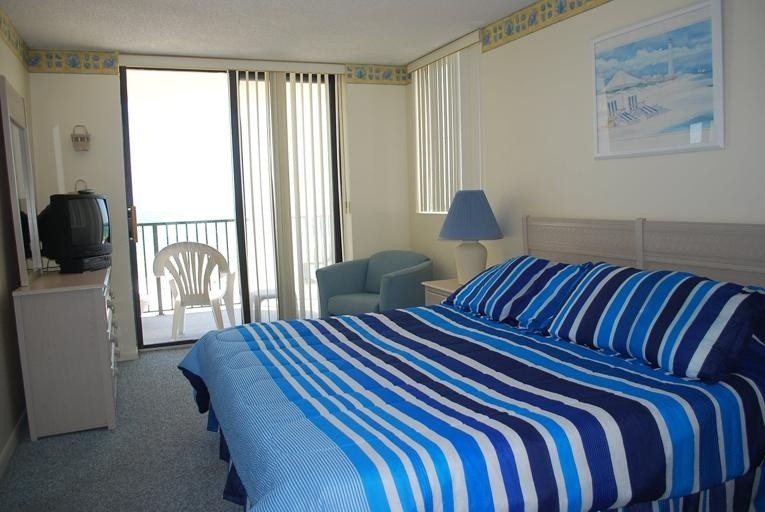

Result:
[420,277,468,307]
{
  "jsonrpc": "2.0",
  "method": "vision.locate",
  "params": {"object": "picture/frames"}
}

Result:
[592,1,725,159]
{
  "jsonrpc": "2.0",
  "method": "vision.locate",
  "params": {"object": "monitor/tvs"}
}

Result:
[38,192,113,275]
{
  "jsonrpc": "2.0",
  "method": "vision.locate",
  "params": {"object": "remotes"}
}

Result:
[77,191,94,194]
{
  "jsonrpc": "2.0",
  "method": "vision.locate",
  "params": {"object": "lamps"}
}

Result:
[436,189,503,285]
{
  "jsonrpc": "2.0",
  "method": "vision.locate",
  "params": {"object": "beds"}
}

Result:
[178,214,764,512]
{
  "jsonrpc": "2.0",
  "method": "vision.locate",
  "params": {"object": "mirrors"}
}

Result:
[0,75,43,283]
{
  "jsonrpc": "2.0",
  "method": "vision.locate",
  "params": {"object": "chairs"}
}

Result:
[153,241,235,338]
[315,248,437,320]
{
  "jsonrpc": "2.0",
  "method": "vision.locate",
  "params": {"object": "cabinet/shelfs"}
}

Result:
[14,264,119,441]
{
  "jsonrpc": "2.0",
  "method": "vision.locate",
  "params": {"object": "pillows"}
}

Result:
[550,261,764,382]
[440,254,597,336]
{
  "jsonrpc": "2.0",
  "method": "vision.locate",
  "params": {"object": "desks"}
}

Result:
[240,288,301,324]
